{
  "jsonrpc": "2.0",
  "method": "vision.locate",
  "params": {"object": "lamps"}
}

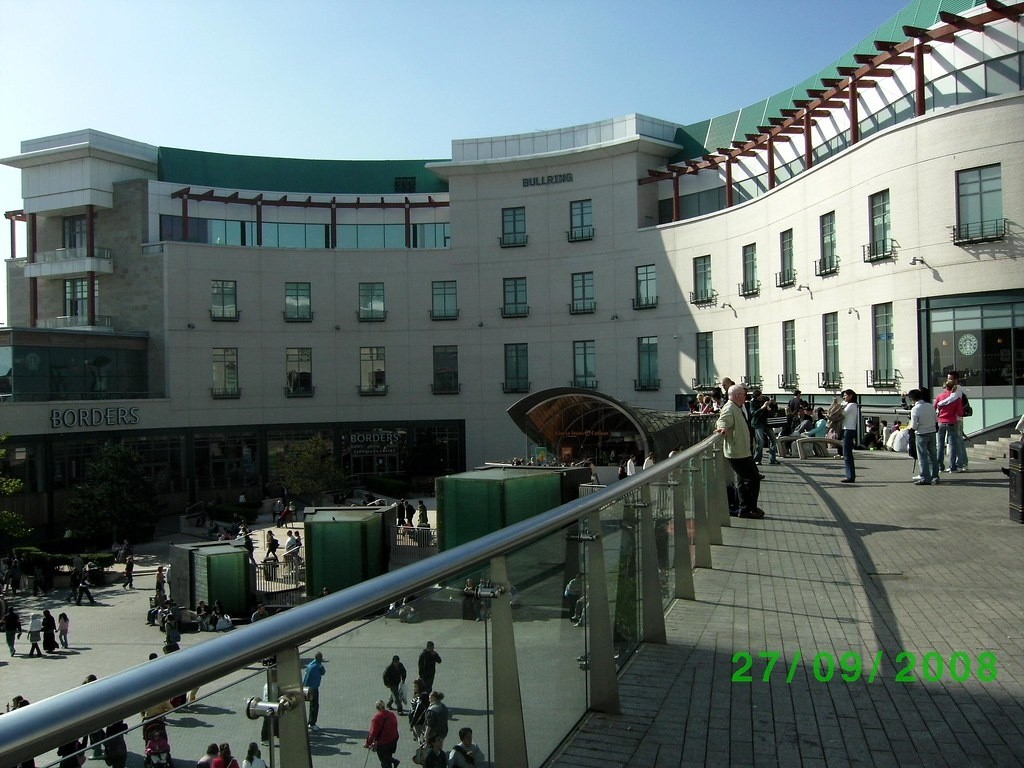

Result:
[721,302,731,308]
[848,307,858,314]
[335,325,340,330]
[910,256,924,265]
[797,285,809,291]
[610,314,618,321]
[187,324,195,329]
[478,322,484,327]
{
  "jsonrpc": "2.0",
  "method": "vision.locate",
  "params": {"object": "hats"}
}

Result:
[804,407,812,411]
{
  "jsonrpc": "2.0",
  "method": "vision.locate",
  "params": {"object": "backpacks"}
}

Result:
[273,539,279,548]
[727,481,738,515]
[961,391,973,416]
[167,622,180,642]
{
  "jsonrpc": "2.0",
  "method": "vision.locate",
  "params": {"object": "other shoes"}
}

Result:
[755,462,762,465]
[914,478,931,485]
[261,741,269,746]
[932,477,940,484]
[397,709,403,715]
[386,705,393,710]
[88,755,103,760]
[145,621,156,626]
[949,467,962,473]
[913,475,922,479]
[573,622,583,627]
[393,759,400,768]
[769,461,780,464]
[840,478,855,482]
[834,455,844,458]
[738,508,765,519]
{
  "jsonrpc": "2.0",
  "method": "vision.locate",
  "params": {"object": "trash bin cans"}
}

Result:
[1008,441,1024,524]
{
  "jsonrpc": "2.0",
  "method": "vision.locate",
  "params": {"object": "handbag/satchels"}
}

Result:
[766,408,787,428]
[216,614,234,630]
[412,742,430,765]
[825,428,839,449]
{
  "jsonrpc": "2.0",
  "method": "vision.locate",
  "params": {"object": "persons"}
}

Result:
[464,578,492,622]
[197,742,267,768]
[319,587,331,599]
[260,652,326,746]
[689,370,969,519]
[145,565,225,645]
[512,452,655,482]
[0,539,135,605]
[0,653,200,768]
[0,594,69,658]
[564,573,590,627]
[364,641,485,768]
[202,490,428,567]
[250,604,269,624]
